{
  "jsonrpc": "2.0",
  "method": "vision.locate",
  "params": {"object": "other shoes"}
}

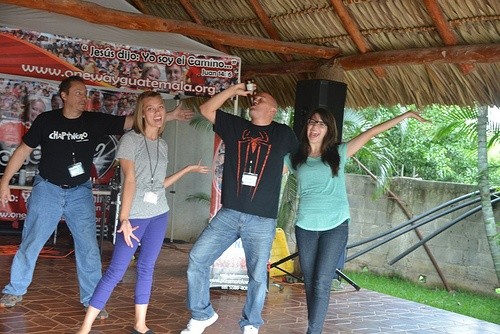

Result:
[180,310,219,334]
[243,325,258,334]
[84,304,109,319]
[132,325,155,334]
[0,294,23,308]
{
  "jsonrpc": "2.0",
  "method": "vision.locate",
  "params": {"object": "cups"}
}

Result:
[19,169,26,186]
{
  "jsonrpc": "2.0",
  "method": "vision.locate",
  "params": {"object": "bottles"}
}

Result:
[245,79,257,107]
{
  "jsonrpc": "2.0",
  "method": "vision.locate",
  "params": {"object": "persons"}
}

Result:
[2,23,239,187]
[282,108,432,334]
[75,90,210,334]
[176,81,302,334]
[0,76,194,319]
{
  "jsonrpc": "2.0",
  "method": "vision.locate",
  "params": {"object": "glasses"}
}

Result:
[307,119,327,127]
